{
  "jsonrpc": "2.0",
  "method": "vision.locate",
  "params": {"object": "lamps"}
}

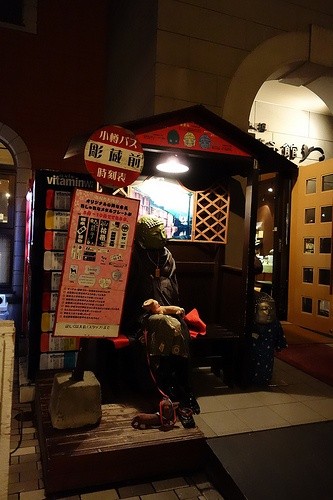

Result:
[156,156,189,173]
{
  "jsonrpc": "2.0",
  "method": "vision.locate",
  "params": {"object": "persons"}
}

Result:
[123,218,200,427]
[254,292,276,324]
